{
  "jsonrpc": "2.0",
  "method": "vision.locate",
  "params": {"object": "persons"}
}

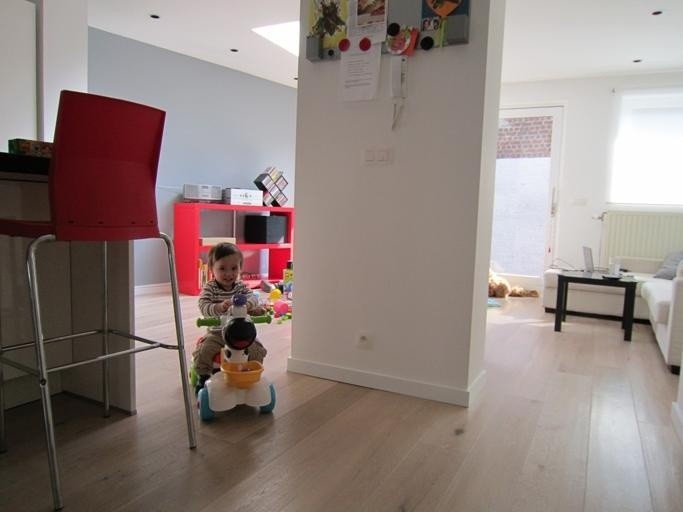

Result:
[192,241,267,396]
[422,18,440,32]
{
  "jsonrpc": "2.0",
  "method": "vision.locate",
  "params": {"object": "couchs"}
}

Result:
[542,256,683,375]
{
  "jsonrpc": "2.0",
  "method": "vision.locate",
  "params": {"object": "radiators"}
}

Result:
[598,202,683,269]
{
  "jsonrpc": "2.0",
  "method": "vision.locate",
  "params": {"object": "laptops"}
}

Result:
[581,244,606,272]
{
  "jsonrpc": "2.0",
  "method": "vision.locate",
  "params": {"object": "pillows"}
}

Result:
[653,252,683,280]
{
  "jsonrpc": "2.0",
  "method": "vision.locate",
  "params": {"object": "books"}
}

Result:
[197,257,215,290]
[487,299,501,308]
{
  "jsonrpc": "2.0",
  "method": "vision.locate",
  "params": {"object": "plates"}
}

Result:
[603,275,622,281]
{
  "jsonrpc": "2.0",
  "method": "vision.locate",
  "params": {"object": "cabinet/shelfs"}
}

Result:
[171,202,295,296]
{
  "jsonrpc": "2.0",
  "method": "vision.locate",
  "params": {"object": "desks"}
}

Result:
[0,152,136,416]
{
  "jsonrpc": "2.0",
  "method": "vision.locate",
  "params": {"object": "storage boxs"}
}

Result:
[222,188,264,206]
[244,214,288,244]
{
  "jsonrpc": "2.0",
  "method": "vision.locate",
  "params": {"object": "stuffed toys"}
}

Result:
[487,268,511,299]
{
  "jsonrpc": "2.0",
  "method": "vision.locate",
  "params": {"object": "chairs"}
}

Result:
[0,89,196,512]
